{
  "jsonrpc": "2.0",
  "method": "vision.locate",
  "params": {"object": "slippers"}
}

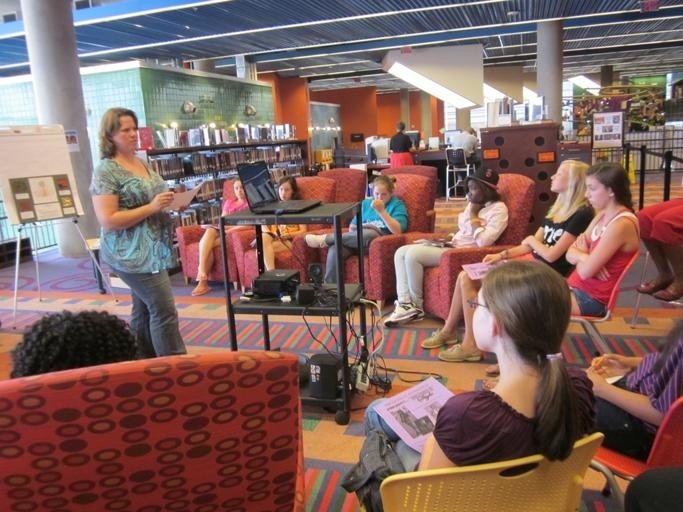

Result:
[191,287,214,296]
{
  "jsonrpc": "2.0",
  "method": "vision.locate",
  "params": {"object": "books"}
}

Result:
[150,123,305,237]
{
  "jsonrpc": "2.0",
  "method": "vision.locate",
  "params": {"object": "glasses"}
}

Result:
[467,295,490,311]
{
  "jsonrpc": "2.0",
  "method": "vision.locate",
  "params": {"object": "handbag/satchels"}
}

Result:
[341,428,408,512]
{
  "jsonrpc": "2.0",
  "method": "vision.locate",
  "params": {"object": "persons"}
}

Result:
[635,198,683,301]
[581,318,683,464]
[421,160,596,361]
[384,167,508,328]
[191,177,256,296]
[389,122,423,168]
[451,128,482,184]
[250,175,307,270]
[88,107,190,360]
[305,175,409,283]
[10,309,147,379]
[481,162,640,391]
[363,260,598,512]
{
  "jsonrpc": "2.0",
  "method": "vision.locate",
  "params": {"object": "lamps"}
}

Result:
[383,44,485,112]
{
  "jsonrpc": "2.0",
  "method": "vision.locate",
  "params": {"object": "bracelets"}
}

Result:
[500,249,512,259]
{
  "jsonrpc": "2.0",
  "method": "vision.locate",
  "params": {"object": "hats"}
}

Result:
[467,168,500,190]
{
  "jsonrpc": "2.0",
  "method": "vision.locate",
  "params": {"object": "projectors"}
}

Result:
[252,269,300,297]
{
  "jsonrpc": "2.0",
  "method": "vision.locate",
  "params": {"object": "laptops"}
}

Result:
[237,161,322,214]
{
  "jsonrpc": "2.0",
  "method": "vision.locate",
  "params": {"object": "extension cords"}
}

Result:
[355,359,371,392]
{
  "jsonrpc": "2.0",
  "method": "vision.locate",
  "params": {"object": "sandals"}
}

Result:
[635,276,683,301]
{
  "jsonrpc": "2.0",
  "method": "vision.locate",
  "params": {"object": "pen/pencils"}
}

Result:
[589,356,604,372]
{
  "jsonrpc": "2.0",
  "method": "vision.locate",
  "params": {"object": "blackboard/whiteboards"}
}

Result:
[0,124,84,226]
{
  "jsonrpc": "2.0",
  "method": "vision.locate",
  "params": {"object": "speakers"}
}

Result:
[306,263,324,284]
[295,285,314,305]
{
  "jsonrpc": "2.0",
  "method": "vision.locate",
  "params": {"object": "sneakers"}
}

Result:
[439,343,483,363]
[384,300,425,328]
[420,327,457,350]
[305,233,329,248]
